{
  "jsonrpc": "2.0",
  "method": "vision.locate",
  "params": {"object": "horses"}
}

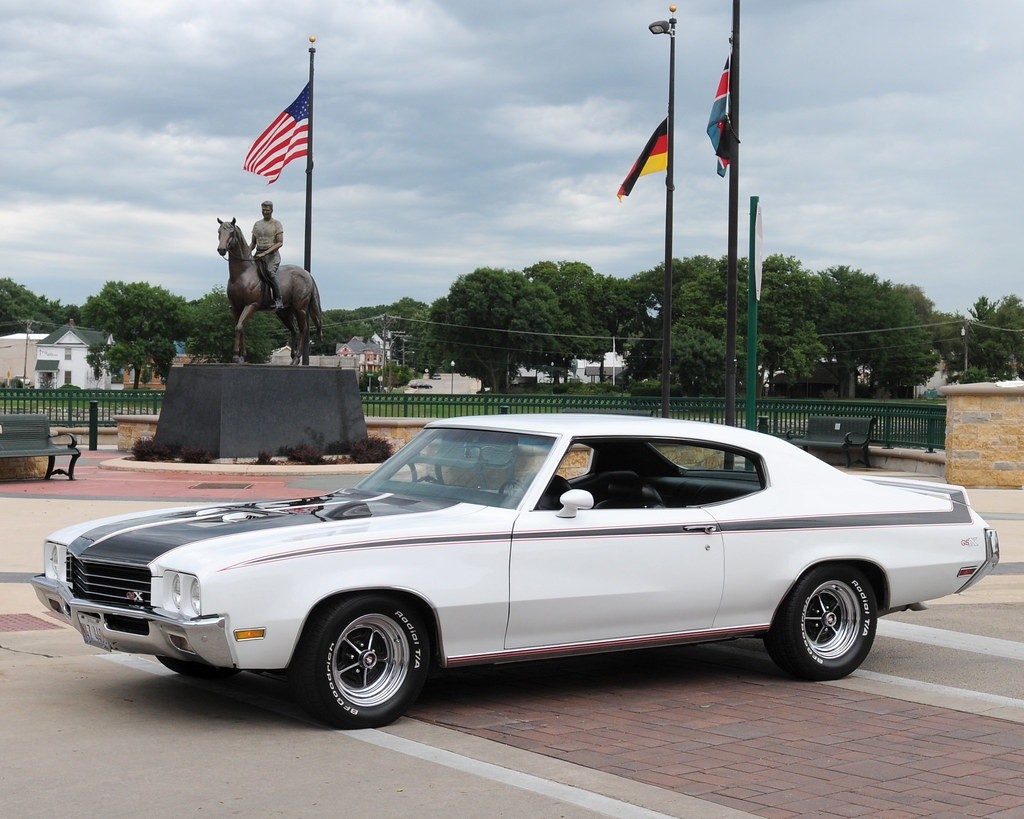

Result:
[217,217,322,365]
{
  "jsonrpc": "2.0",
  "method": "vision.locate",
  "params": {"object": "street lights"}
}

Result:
[450,360,455,393]
[649,17,678,417]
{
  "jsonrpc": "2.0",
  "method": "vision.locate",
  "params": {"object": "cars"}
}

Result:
[13,375,30,385]
[2,379,23,388]
[30,413,1002,732]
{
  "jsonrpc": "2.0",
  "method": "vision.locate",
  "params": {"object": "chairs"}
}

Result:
[536,471,665,509]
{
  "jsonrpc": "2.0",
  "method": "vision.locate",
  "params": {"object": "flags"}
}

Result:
[243,82,311,184]
[707,54,731,177]
[618,116,668,201]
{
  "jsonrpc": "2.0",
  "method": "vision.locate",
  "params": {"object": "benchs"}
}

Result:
[0,414,81,480]
[644,475,762,508]
[787,415,877,468]
[407,441,518,493]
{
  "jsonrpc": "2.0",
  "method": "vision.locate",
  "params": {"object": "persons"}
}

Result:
[248,200,284,308]
